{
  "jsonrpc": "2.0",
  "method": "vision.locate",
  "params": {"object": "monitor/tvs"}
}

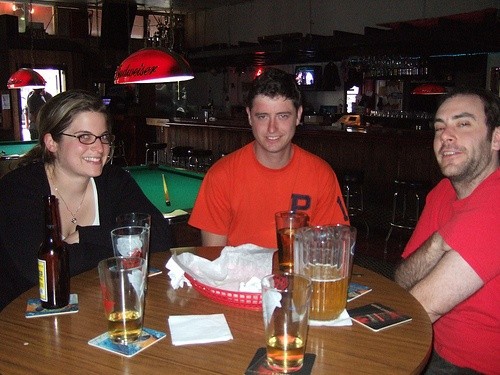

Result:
[292,65,321,91]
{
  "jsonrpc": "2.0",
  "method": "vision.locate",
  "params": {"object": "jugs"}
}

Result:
[292,224,351,322]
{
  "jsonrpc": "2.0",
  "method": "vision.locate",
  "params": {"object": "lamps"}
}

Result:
[7,0,47,90]
[113,0,196,84]
[411,83,448,95]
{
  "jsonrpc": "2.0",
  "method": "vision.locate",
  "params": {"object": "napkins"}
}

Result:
[166,243,353,347]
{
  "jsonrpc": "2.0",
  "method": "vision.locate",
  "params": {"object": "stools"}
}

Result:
[338,170,370,239]
[384,177,430,253]
[145,142,228,172]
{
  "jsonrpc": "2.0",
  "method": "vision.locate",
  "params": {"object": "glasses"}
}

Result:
[60,132,115,144]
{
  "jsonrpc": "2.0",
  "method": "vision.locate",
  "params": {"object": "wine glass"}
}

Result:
[365,54,431,76]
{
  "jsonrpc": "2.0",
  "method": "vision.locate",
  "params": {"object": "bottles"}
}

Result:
[37,195,71,309]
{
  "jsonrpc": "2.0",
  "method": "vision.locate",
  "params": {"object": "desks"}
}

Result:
[0,140,39,178]
[122,161,204,248]
[0,245,433,375]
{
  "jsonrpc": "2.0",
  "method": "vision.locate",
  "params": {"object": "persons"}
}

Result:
[27,86,53,141]
[187,67,351,246]
[0,89,175,309]
[394,85,500,375]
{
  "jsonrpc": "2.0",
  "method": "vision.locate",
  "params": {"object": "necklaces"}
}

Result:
[48,164,84,224]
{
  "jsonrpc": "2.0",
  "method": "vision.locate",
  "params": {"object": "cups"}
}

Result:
[275,210,308,273]
[111,226,150,295]
[261,273,312,372]
[364,106,409,118]
[97,256,147,345]
[343,225,357,276]
[115,212,151,236]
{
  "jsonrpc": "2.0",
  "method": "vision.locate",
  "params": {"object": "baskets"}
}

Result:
[185,273,289,310]
